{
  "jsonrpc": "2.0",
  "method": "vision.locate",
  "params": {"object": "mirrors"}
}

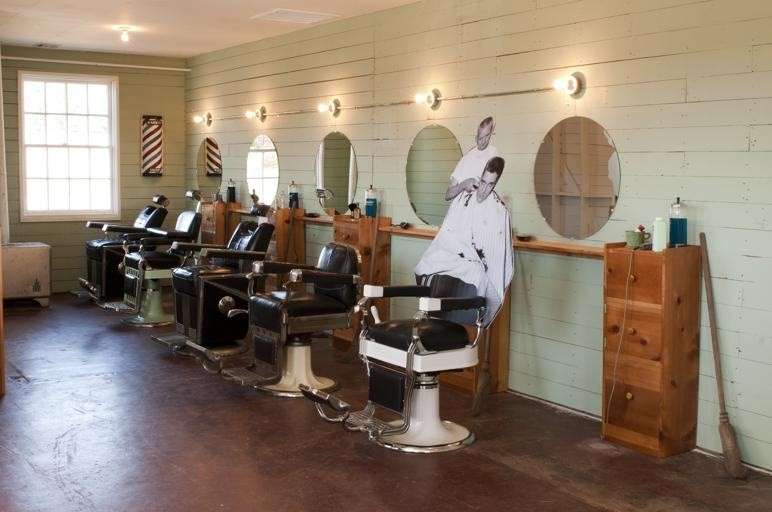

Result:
[197,137,222,203]
[246,134,279,206]
[533,116,621,240]
[406,125,463,228]
[314,132,357,217]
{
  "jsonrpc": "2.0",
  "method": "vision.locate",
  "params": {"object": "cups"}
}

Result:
[624,230,650,246]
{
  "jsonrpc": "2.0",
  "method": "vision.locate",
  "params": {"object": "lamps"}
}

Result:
[194,111,212,127]
[552,76,582,96]
[415,91,438,108]
[244,107,267,121]
[119,27,131,41]
[319,101,338,115]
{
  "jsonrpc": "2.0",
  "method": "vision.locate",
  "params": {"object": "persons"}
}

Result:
[444,115,501,203]
[414,156,516,326]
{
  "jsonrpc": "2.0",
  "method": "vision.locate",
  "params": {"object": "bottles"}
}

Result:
[288,180,299,209]
[227,178,235,203]
[273,190,286,211]
[354,202,361,218]
[365,185,378,217]
[651,217,668,253]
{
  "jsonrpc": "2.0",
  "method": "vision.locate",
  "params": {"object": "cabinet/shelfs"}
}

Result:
[600,242,702,457]
